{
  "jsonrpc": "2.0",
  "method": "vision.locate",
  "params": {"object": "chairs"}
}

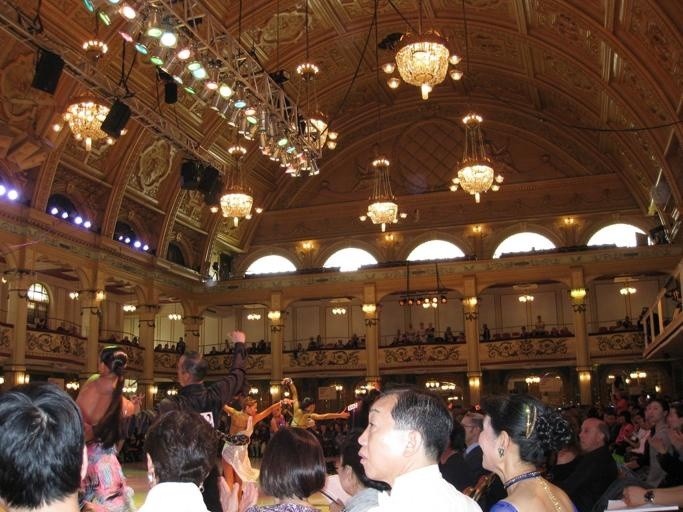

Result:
[453,448,654,512]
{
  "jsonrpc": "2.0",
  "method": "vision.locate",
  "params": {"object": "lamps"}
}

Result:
[13,370,29,386]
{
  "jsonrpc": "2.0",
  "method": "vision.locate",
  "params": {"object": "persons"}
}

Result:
[0,306,680,511]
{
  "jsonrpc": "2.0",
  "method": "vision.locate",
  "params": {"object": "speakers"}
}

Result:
[100,100,131,137]
[31,50,64,94]
[181,161,224,205]
[165,83,177,103]
